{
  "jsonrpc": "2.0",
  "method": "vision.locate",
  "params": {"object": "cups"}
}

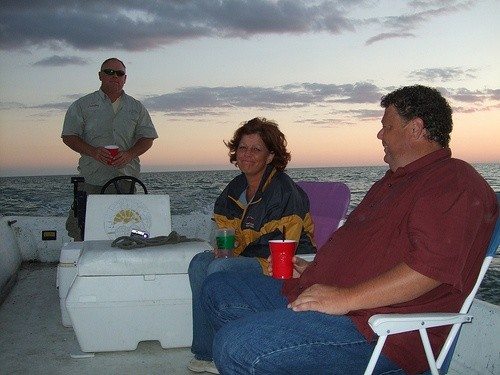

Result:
[268,240,296,280]
[104,145,119,166]
[216,228,236,258]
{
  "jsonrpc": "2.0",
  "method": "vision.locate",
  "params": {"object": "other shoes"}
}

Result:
[187,356,220,374]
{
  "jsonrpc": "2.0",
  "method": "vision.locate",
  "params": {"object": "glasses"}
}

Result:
[102,69,125,76]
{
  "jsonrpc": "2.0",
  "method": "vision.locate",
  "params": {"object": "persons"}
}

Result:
[60,58,159,194]
[203,84,498,375]
[188,118,317,375]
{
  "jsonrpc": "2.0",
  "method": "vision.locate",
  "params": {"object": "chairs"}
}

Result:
[275,181,351,263]
[57,194,215,351]
[362,190,500,375]
